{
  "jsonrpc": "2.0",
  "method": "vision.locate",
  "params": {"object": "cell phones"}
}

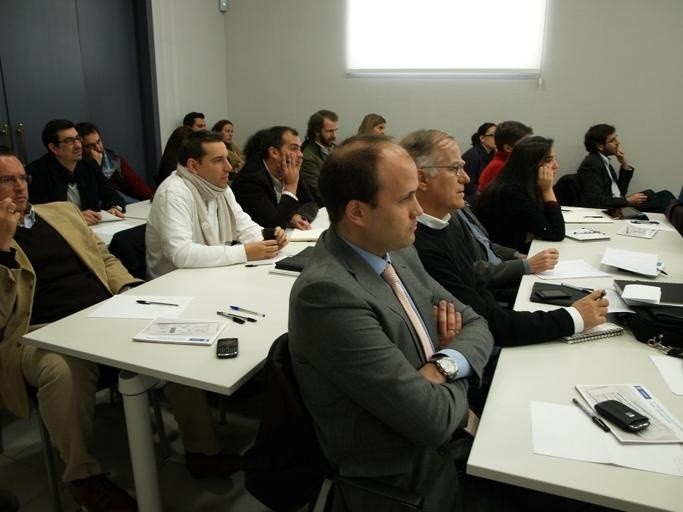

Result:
[595,399,651,433]
[263,228,277,241]
[217,338,238,358]
[535,289,572,299]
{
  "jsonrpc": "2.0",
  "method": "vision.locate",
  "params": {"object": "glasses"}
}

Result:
[421,164,463,176]
[53,135,81,143]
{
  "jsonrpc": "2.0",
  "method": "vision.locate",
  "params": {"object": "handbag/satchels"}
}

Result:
[627,306,683,358]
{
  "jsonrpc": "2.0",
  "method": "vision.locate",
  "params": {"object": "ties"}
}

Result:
[381,263,479,436]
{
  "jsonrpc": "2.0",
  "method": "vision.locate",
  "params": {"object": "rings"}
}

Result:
[6,209,15,214]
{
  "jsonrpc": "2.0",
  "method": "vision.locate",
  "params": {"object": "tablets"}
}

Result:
[603,206,641,219]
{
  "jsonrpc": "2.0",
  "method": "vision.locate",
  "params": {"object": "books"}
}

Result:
[564,227,611,242]
[131,314,229,347]
[613,279,683,308]
[559,320,625,344]
[528,281,596,308]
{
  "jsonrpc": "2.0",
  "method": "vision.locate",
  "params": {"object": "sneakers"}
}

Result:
[67,476,138,512]
[184,449,242,480]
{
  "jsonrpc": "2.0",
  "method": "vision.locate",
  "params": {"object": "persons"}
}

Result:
[0,149,234,512]
[76,122,150,206]
[396,127,615,345]
[154,111,387,231]
[462,121,565,255]
[21,119,125,222]
[570,123,676,213]
[141,128,292,280]
[283,132,541,511]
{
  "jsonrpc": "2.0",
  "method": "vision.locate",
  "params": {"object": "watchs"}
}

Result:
[425,355,460,382]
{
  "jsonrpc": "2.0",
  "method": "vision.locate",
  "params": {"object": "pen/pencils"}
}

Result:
[585,216,604,219]
[630,221,660,224]
[136,300,181,306]
[245,261,273,267]
[215,305,266,325]
[573,395,609,434]
[657,268,670,276]
[562,210,571,211]
[573,230,602,234]
[561,282,596,293]
